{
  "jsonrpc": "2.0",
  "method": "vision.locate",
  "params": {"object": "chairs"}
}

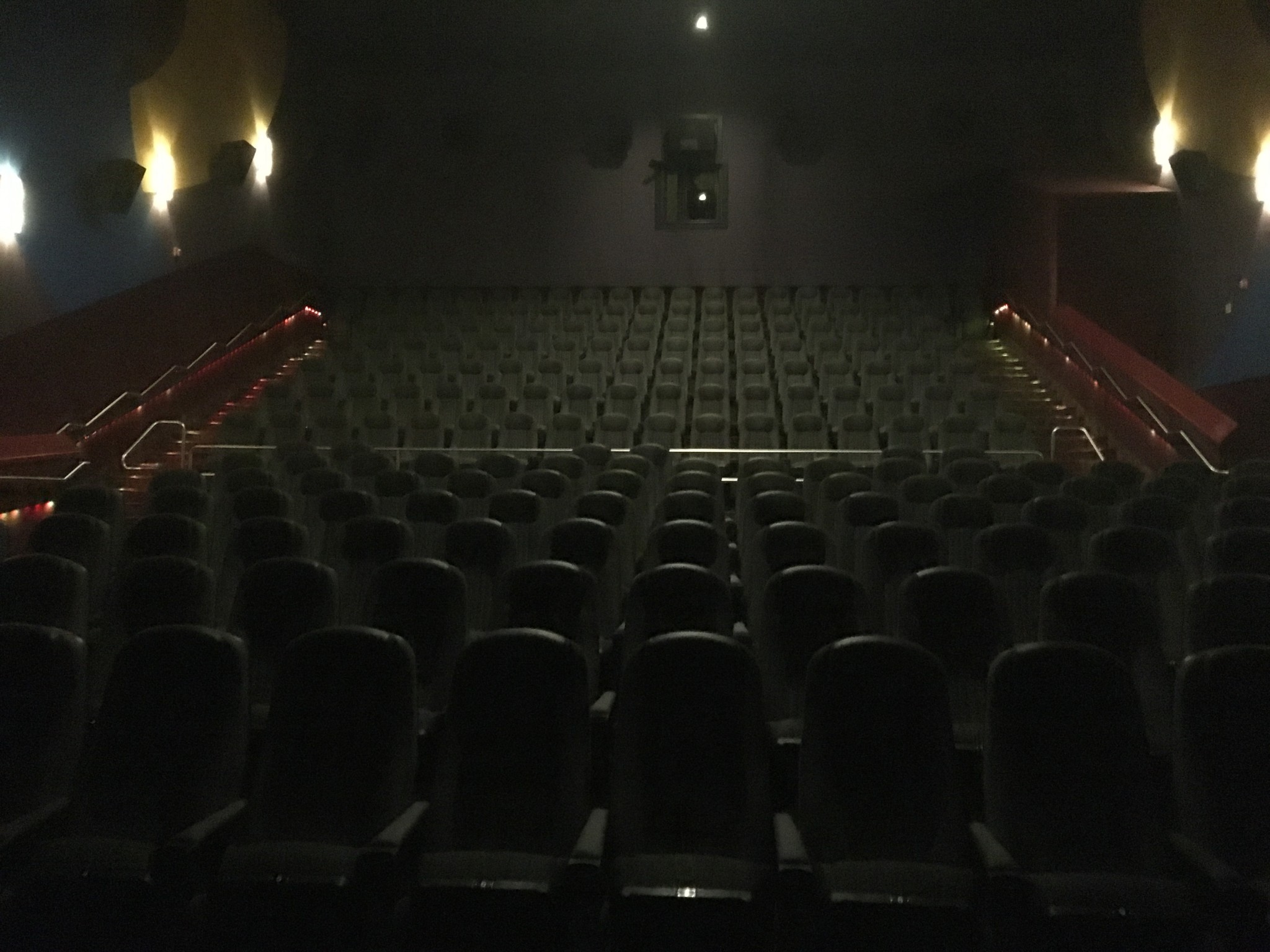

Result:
[2,271,1270,951]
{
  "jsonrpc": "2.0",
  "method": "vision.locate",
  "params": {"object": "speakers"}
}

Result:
[208,140,256,189]
[1169,150,1212,197]
[80,158,147,219]
